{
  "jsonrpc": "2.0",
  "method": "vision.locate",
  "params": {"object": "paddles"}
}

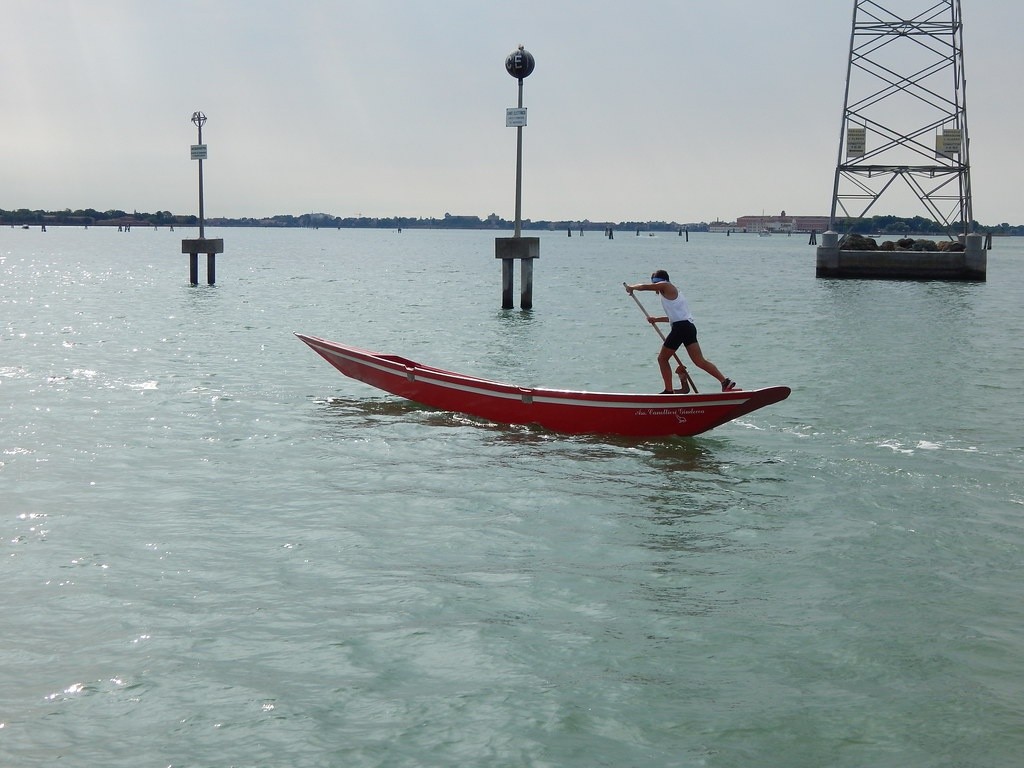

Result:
[621,283,699,393]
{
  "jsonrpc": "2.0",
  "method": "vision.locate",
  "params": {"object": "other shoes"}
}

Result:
[722,378,736,392]
[659,389,674,394]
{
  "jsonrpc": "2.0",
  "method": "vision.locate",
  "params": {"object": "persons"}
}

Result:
[626,269,737,394]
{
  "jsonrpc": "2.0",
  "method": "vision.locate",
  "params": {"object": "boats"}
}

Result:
[292,330,792,442]
